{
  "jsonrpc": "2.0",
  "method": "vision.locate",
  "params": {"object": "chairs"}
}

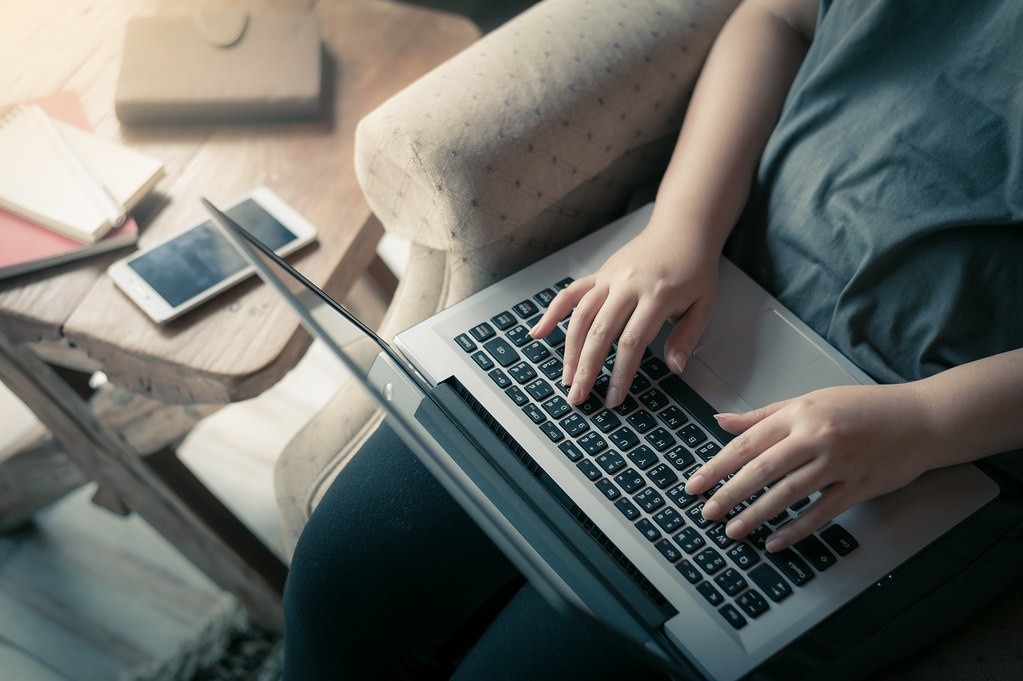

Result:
[273,2,740,550]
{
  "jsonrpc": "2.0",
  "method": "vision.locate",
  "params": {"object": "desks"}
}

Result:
[0,0,482,636]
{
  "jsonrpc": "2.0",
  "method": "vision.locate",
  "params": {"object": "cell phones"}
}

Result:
[108,186,318,327]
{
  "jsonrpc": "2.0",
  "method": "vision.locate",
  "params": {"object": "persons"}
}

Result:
[285,1,1023,681]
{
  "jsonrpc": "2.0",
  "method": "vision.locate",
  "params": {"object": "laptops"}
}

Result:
[201,196,1002,681]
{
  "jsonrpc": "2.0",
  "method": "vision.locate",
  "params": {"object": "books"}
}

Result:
[114,8,323,122]
[0,87,166,278]
